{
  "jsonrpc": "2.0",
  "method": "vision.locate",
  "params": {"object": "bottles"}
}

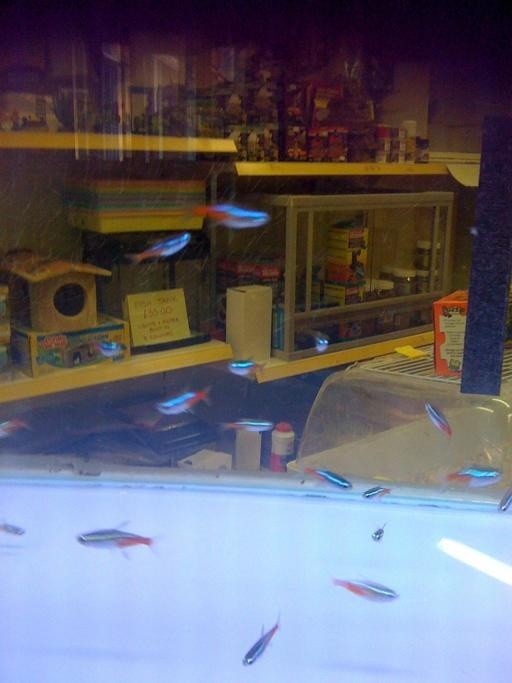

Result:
[271,420,296,474]
